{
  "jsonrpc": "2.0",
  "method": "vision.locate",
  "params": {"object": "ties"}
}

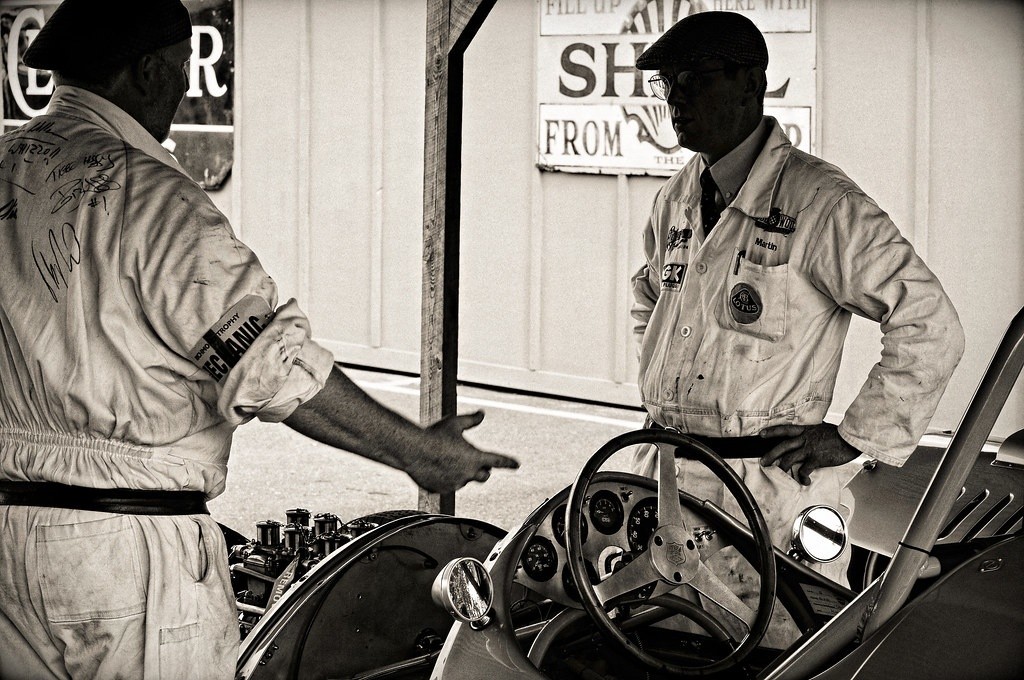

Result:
[694,169,726,237]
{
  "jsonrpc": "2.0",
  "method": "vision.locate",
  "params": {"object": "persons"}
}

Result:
[0,0,521,680]
[628,12,967,589]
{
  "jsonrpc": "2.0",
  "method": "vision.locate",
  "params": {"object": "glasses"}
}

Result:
[649,61,738,100]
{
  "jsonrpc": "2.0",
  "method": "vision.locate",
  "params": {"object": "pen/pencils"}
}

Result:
[733,249,745,275]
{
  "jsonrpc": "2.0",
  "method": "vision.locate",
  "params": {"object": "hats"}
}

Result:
[23,0,193,75]
[639,9,770,71]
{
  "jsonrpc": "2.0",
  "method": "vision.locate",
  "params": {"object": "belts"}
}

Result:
[0,477,212,515]
[647,417,786,459]
